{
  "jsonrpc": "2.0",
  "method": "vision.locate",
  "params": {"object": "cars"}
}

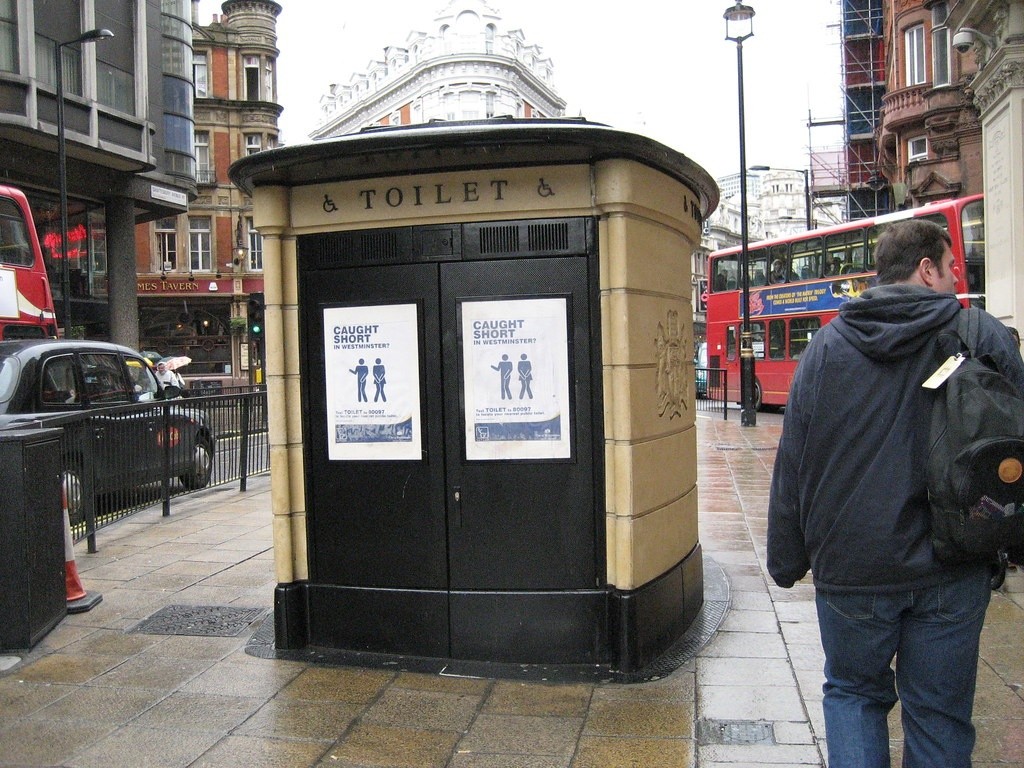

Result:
[694,342,708,400]
[0,336,216,529]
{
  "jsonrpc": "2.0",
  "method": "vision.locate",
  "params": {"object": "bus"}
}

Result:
[704,191,985,414]
[0,183,70,398]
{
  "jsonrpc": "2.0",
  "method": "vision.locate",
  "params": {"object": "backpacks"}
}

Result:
[922,307,1024,573]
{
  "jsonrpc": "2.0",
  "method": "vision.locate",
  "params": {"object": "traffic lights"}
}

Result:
[247,290,264,340]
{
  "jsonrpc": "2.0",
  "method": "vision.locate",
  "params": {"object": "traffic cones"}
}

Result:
[62,480,105,615]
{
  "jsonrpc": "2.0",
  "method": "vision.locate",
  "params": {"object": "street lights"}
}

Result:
[748,165,811,231]
[55,28,116,341]
[720,0,757,427]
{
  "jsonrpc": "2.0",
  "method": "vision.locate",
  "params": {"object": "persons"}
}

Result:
[768,219,1024,768]
[769,259,793,283]
[824,251,841,276]
[173,370,185,389]
[154,362,181,390]
[716,270,728,291]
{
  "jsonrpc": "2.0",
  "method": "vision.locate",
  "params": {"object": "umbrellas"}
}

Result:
[156,356,192,370]
[140,351,163,359]
